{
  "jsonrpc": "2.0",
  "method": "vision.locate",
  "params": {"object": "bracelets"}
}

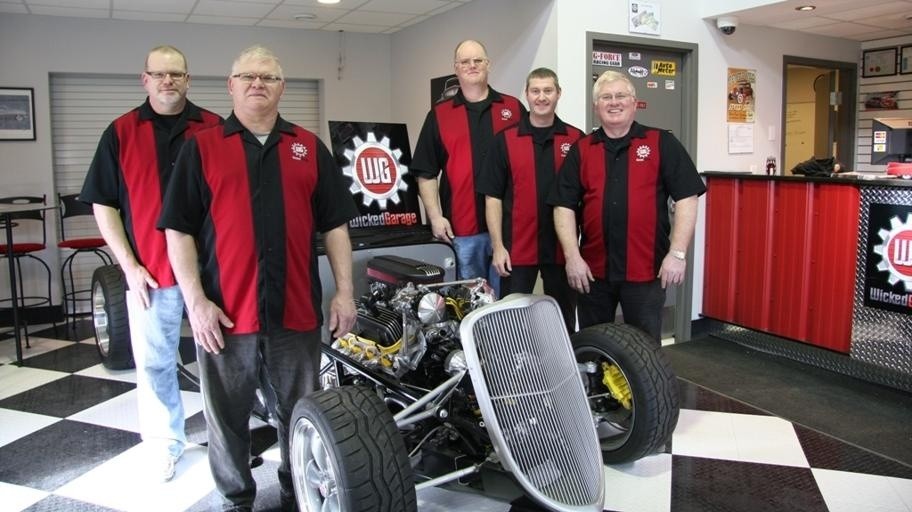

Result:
[668,249,687,261]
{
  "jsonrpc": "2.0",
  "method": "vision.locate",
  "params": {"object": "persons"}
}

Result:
[476,67,587,334]
[154,44,358,511]
[543,70,709,348]
[408,39,528,279]
[77,45,227,484]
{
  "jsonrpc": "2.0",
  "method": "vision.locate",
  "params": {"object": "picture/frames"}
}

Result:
[0,87,36,141]
[862,47,898,78]
[900,45,912,75]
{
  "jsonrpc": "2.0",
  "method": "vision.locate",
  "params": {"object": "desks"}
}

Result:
[0,204,58,367]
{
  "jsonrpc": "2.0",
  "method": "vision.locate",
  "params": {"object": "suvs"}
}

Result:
[88,119,682,510]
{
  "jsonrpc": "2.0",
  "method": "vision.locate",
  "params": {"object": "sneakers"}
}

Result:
[162,448,185,480]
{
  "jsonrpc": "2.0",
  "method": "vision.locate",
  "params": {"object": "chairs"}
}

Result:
[58,192,113,329]
[0,194,59,349]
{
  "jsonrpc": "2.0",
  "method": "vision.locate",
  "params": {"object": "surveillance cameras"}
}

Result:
[717,16,739,35]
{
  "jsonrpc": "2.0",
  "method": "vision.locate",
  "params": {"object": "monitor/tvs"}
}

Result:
[871,118,912,165]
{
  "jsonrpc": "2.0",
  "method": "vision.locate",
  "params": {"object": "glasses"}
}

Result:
[146,70,185,80]
[600,92,632,100]
[456,58,486,65]
[233,72,282,84]
